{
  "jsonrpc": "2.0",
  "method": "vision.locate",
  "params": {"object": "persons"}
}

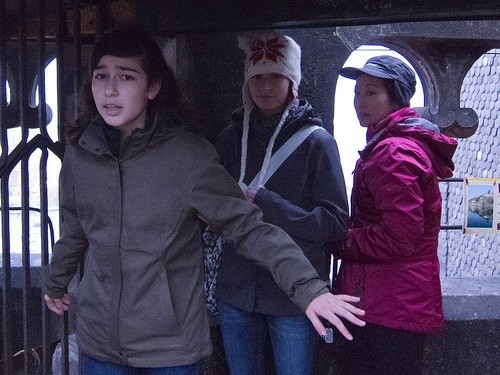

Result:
[43,29,366,375]
[213,30,350,375]
[328,56,458,375]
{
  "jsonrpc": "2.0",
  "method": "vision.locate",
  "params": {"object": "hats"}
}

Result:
[237,30,301,201]
[339,55,416,107]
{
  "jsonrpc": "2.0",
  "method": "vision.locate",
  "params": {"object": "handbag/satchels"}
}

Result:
[201,224,227,313]
